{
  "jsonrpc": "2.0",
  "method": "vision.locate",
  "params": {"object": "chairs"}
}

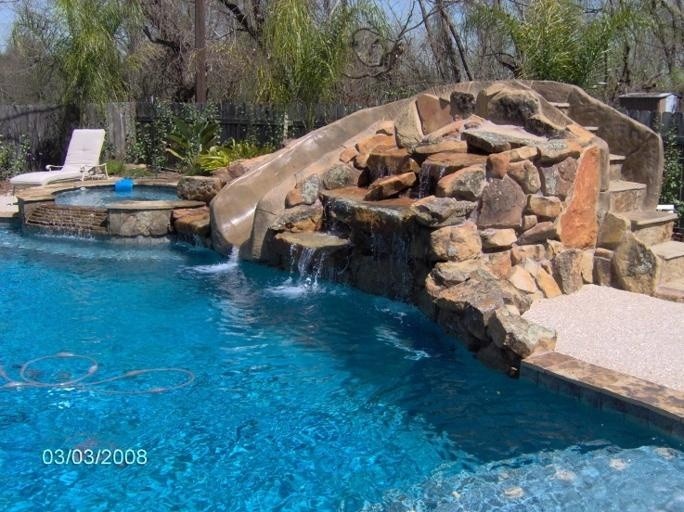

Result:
[9,128,109,196]
